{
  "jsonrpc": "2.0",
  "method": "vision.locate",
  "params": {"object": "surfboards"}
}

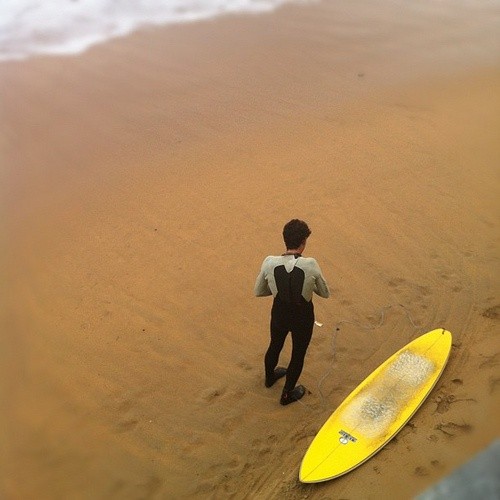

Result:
[298,327,454,483]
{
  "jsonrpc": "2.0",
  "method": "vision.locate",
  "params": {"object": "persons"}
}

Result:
[253,219,330,405]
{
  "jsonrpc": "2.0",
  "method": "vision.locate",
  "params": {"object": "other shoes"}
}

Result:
[281,385,305,405]
[266,367,286,387]
